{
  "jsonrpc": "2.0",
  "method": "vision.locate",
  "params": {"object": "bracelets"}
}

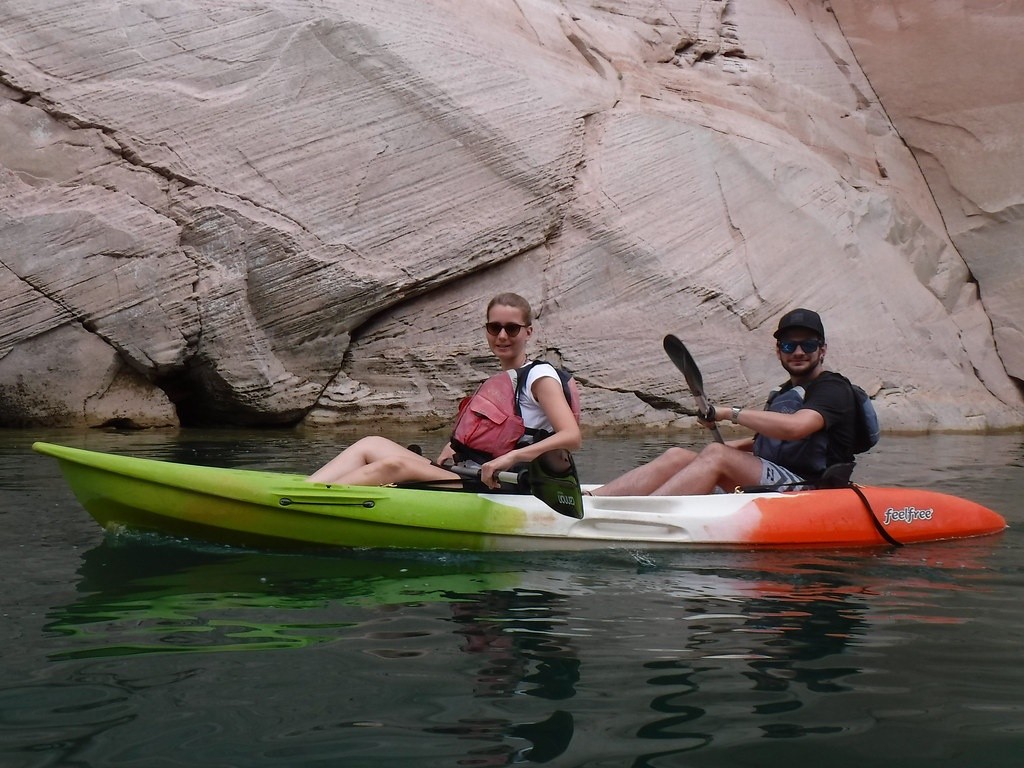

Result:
[732,406,741,424]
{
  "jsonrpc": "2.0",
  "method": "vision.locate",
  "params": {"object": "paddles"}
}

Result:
[661,332,727,447]
[436,448,585,518]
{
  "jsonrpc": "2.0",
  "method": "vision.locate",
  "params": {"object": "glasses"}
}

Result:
[779,339,821,354]
[484,321,529,337]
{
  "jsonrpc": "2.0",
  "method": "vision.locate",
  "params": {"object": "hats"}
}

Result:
[773,308,825,341]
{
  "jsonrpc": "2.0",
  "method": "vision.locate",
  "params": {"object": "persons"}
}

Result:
[583,308,857,496]
[306,292,581,497]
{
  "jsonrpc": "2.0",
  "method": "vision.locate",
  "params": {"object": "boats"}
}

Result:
[31,437,1011,554]
